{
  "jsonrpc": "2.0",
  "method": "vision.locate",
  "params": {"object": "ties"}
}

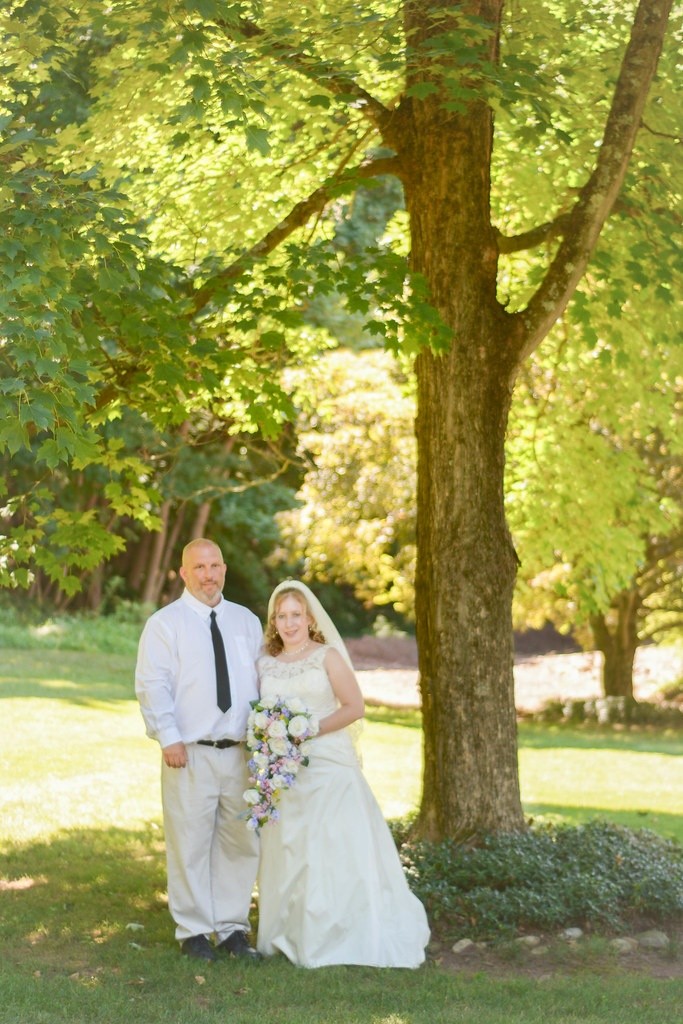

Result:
[209,610,232,714]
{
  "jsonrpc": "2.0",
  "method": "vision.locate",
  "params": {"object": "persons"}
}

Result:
[252,576,433,969]
[134,538,268,962]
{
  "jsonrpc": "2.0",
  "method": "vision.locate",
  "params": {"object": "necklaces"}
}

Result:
[282,639,311,656]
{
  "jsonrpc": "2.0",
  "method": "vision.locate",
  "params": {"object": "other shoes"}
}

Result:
[182,934,215,960]
[224,930,262,960]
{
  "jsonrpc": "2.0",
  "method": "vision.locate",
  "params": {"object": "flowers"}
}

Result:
[240,693,319,839]
